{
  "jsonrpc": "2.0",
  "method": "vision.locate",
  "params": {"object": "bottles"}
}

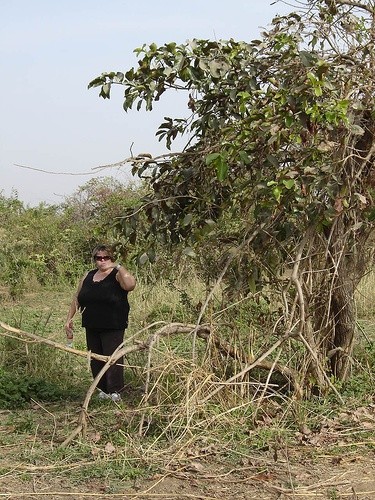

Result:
[67,320,74,349]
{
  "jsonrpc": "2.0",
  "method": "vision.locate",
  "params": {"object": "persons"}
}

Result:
[64,244,136,402]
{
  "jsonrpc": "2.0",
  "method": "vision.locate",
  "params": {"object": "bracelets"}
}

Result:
[116,264,121,270]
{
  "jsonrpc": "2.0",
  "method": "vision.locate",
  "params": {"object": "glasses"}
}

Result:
[95,255,112,261]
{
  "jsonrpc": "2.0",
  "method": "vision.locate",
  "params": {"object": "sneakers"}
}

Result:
[97,392,121,401]
